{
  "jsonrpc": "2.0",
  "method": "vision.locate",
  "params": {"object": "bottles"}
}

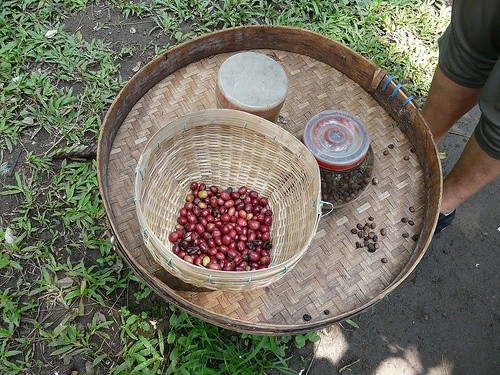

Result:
[292,109,376,211]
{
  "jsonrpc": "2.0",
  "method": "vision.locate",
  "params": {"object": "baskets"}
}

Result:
[132,109,333,293]
[96,25,442,339]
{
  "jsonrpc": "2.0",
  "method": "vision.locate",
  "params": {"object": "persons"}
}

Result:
[419,0,500,238]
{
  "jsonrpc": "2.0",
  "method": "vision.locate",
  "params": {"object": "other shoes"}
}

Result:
[432,209,456,239]
